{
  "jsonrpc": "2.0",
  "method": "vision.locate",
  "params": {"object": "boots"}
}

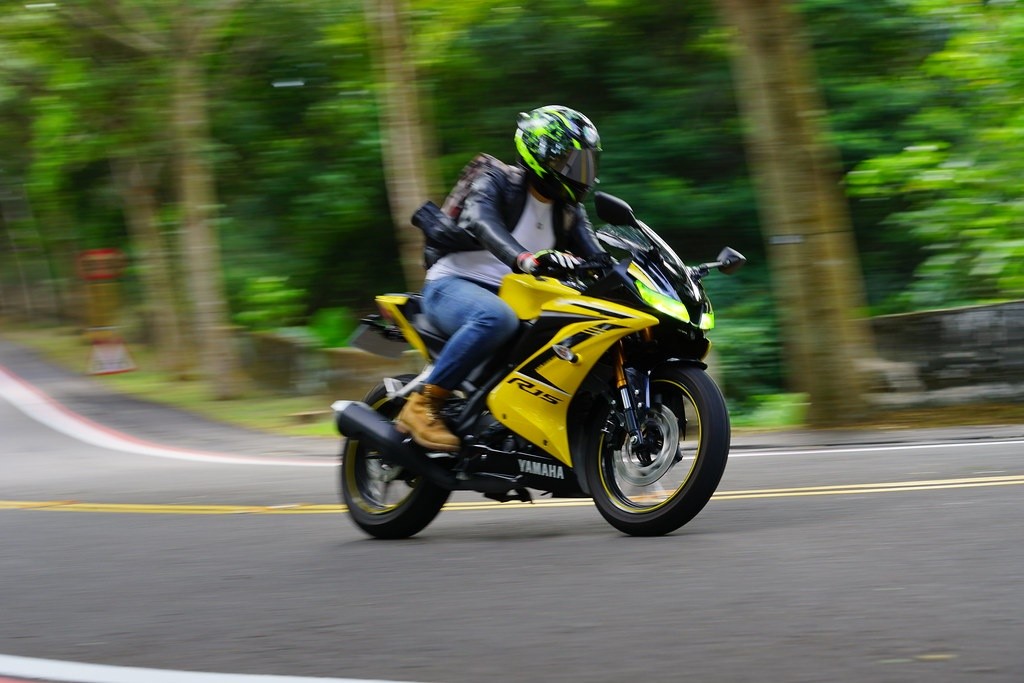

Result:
[394,382,460,450]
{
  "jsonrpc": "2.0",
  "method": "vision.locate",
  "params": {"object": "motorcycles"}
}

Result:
[329,194,750,538]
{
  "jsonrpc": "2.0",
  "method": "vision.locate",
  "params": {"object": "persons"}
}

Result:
[395,105,620,448]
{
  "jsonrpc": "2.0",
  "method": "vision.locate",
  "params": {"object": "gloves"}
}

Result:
[522,249,580,282]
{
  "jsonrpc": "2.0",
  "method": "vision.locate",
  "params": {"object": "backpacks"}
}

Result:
[420,150,521,272]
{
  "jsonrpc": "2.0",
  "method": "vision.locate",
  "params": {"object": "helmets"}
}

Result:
[514,104,603,207]
